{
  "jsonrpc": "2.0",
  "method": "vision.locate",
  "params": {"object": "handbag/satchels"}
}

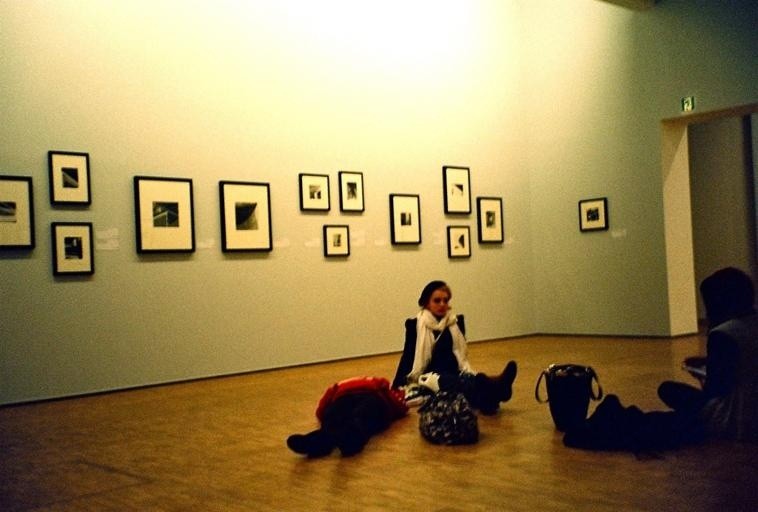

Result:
[417,388,479,443]
[534,363,602,432]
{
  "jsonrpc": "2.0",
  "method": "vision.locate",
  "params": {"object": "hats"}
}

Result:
[418,280,444,306]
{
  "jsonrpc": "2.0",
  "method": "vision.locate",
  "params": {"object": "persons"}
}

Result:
[562,266,757,457]
[392,279,518,417]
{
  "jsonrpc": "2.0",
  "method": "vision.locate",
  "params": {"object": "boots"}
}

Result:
[475,361,516,417]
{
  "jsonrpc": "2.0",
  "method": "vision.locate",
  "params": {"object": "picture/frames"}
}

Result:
[577,195,609,234]
[1,172,39,254]
[217,178,276,254]
[49,220,97,278]
[46,149,95,209]
[337,169,367,215]
[446,224,472,261]
[133,174,198,257]
[441,164,473,216]
[387,191,423,246]
[321,222,352,259]
[298,172,333,213]
[475,195,505,246]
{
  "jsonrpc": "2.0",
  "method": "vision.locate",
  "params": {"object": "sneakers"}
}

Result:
[288,433,331,457]
